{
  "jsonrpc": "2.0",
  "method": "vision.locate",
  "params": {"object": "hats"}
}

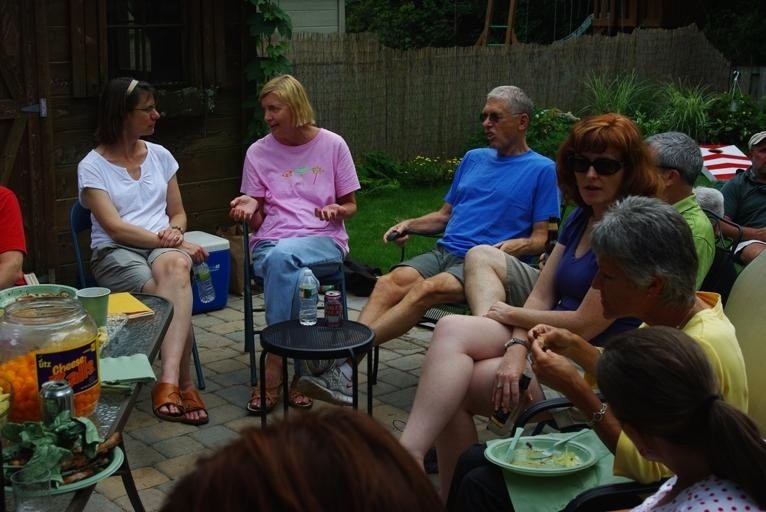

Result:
[748,131,766,147]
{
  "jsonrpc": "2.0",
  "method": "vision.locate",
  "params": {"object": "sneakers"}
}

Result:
[295,352,337,373]
[296,365,356,406]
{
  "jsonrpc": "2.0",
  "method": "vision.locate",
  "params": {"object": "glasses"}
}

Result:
[478,111,521,121]
[569,156,626,176]
[132,104,153,113]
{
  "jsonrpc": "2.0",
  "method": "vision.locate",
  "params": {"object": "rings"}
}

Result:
[496,382,503,390]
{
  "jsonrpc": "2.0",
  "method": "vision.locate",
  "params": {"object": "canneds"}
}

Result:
[324,290,343,329]
[38,379,75,428]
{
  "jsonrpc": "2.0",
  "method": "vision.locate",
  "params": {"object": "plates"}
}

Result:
[2,439,125,496]
[483,435,598,477]
[0,284,79,309]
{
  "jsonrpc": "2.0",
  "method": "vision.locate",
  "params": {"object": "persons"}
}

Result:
[720,131,766,267]
[456,128,719,316]
[147,400,448,512]
[225,72,364,415]
[76,76,213,425]
[390,112,677,512]
[291,82,566,407]
[442,188,750,512]
[0,185,29,292]
[589,322,766,512]
[691,178,737,308]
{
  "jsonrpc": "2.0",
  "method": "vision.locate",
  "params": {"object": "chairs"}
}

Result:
[69,201,207,393]
[698,209,743,294]
[509,394,672,510]
[371,196,568,387]
[242,213,348,387]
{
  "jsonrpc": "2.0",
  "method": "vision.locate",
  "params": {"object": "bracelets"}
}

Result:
[503,338,532,350]
[166,223,187,237]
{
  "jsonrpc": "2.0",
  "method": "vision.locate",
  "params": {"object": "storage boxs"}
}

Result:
[182,230,232,314]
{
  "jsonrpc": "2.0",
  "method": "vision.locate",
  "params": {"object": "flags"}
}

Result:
[693,140,755,185]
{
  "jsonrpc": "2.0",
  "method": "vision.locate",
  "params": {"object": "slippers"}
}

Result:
[150,382,185,421]
[289,376,314,410]
[182,388,209,425]
[247,378,285,412]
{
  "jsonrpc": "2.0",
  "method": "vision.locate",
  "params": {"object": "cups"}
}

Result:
[75,286,111,328]
[10,468,51,511]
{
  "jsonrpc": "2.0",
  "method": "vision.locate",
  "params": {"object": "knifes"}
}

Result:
[505,426,524,464]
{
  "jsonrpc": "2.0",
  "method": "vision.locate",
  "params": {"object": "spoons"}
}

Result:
[528,427,591,460]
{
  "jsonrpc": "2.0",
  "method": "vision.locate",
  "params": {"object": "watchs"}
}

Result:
[584,403,609,428]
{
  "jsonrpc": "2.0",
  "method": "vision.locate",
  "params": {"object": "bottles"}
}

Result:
[191,260,217,306]
[298,269,319,327]
[486,369,533,435]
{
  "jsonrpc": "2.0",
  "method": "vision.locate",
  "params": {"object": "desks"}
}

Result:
[0,291,175,512]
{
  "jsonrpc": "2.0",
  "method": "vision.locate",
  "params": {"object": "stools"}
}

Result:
[259,318,374,432]
